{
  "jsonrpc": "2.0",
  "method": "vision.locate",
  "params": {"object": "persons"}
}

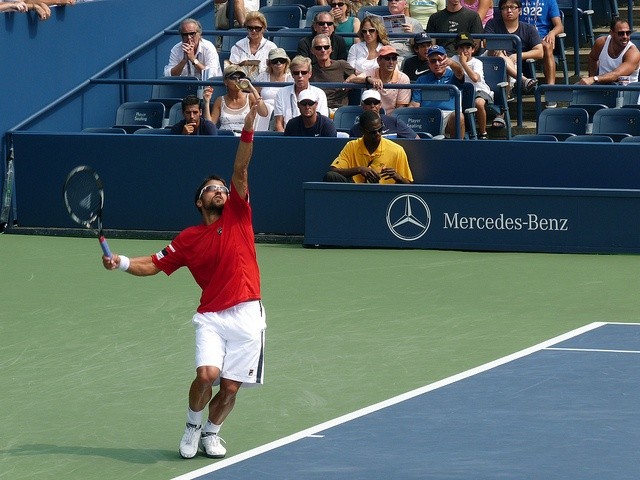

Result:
[0,0,28,13]
[349,45,411,115]
[408,45,465,140]
[347,15,389,76]
[309,33,360,113]
[403,32,432,81]
[322,112,414,185]
[450,32,495,140]
[203,64,268,130]
[426,0,483,57]
[483,0,545,102]
[25,0,51,19]
[215,0,260,29]
[170,95,217,136]
[101,97,267,460]
[252,48,294,131]
[42,0,74,7]
[387,0,425,71]
[519,0,564,108]
[460,0,494,29]
[274,56,328,133]
[578,18,640,85]
[328,0,360,49]
[283,90,337,137]
[349,89,420,139]
[297,11,348,61]
[162,18,223,99]
[229,11,278,93]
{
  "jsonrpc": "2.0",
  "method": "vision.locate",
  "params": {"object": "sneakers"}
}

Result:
[199,432,227,459]
[545,101,557,109]
[179,423,201,459]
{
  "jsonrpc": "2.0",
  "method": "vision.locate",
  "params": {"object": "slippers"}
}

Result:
[492,117,506,129]
[525,79,538,92]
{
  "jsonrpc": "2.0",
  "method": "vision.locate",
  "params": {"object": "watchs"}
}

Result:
[191,58,199,66]
[593,74,600,84]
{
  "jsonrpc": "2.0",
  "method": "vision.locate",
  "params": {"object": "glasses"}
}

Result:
[362,29,375,33]
[430,59,443,63]
[502,5,520,10]
[612,31,632,36]
[230,77,244,80]
[363,100,380,105]
[315,46,330,50]
[182,32,196,39]
[300,101,316,106]
[318,22,333,26]
[363,127,383,135]
[381,56,397,61]
[330,2,344,8]
[271,60,287,64]
[293,71,309,75]
[247,26,263,32]
[199,185,229,200]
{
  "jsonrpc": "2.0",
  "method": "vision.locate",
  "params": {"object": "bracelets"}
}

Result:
[31,3,36,9]
[365,75,371,82]
[13,1,19,5]
[118,256,131,273]
[240,128,254,143]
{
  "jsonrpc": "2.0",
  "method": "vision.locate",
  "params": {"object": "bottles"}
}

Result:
[235,80,250,91]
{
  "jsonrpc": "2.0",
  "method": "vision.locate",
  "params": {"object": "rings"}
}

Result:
[43,15,47,19]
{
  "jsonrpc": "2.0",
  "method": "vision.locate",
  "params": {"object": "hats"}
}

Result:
[362,90,381,100]
[225,65,246,78]
[299,90,319,102]
[414,32,433,43]
[378,46,400,56]
[268,48,289,60]
[456,33,474,48]
[428,45,446,55]
